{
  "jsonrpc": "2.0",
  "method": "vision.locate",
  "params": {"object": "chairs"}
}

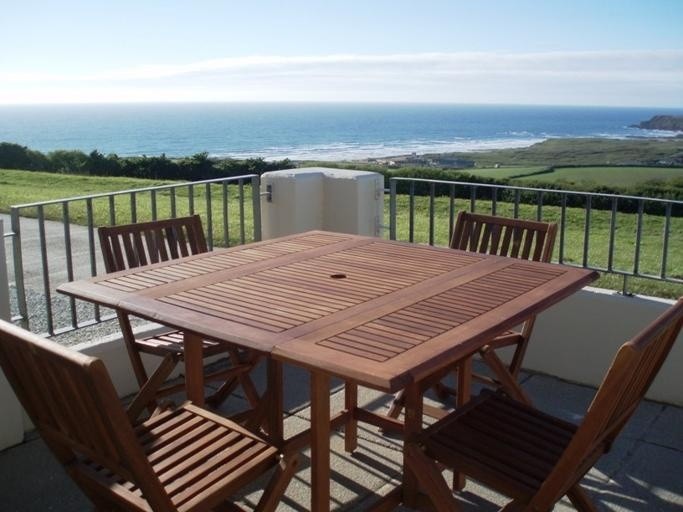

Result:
[401,298,683,511]
[94,213,269,451]
[383,212,567,487]
[0,318,301,511]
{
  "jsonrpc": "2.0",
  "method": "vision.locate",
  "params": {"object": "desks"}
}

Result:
[57,228,595,511]
